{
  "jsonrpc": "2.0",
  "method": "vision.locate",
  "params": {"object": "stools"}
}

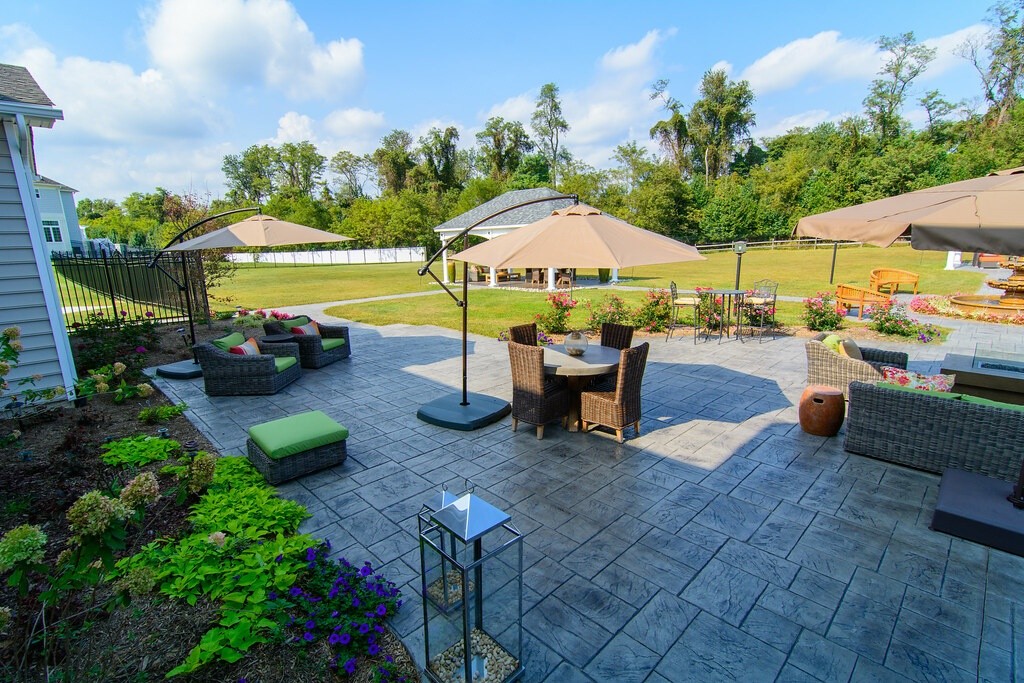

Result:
[800,385,845,435]
[247,411,349,486]
[665,279,779,344]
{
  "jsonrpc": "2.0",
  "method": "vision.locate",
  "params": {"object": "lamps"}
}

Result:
[159,428,198,456]
[236,306,242,314]
[733,242,747,257]
[176,327,189,346]
[4,401,26,419]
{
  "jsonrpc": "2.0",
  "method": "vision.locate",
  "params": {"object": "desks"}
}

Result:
[700,290,747,344]
[537,345,623,432]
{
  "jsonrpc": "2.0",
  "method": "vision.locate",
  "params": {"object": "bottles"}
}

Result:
[564,329,588,355]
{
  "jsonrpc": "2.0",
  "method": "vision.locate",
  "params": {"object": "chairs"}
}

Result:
[507,323,650,441]
[477,265,573,288]
[835,267,919,321]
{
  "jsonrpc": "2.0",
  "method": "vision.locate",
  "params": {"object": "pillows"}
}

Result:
[292,320,320,335]
[231,338,260,356]
[821,334,1024,412]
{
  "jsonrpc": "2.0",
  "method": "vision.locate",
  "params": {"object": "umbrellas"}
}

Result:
[787,161,1023,257]
[147,206,356,364]
[416,193,709,404]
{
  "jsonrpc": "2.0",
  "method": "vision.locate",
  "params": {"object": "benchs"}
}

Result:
[978,254,1006,269]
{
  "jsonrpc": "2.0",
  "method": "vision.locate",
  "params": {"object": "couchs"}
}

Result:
[805,331,908,401]
[843,379,1024,482]
[192,315,351,396]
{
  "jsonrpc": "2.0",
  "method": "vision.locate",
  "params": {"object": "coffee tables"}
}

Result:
[259,334,296,343]
